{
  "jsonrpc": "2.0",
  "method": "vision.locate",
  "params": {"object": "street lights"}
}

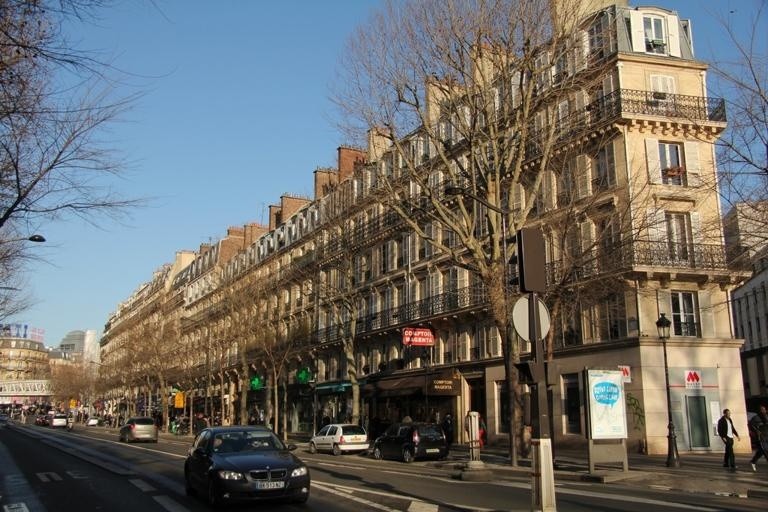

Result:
[655,313,681,467]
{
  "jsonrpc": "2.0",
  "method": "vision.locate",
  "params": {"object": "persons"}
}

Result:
[270,412,275,433]
[441,412,455,453]
[717,408,741,469]
[476,413,489,450]
[249,412,256,424]
[103,410,112,428]
[746,404,768,472]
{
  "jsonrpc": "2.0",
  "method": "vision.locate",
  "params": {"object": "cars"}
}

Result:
[309,424,370,456]
[184,426,310,512]
[120,416,158,443]
[85,416,98,425]
[372,422,448,463]
[35,412,69,429]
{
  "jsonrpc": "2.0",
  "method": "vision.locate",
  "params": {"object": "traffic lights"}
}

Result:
[520,232,548,297]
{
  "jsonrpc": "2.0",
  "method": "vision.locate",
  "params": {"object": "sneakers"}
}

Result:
[749,460,757,471]
[723,463,738,468]
